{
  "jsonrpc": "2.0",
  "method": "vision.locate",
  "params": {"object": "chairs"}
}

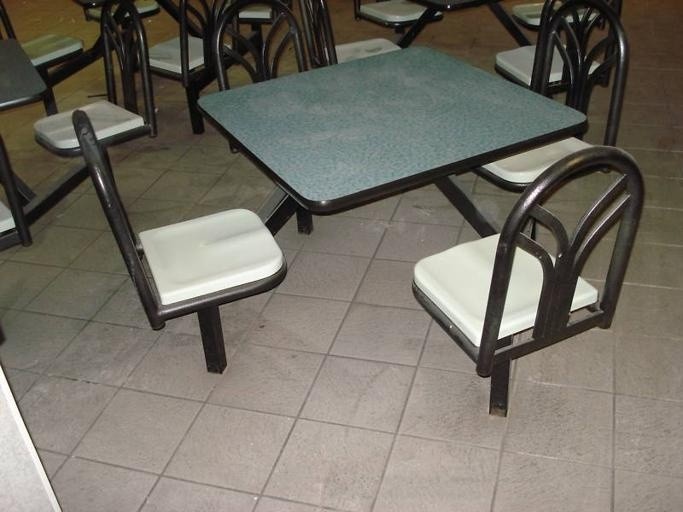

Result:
[24,0,647,417]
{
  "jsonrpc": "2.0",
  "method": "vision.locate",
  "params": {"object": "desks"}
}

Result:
[0,40,47,250]
